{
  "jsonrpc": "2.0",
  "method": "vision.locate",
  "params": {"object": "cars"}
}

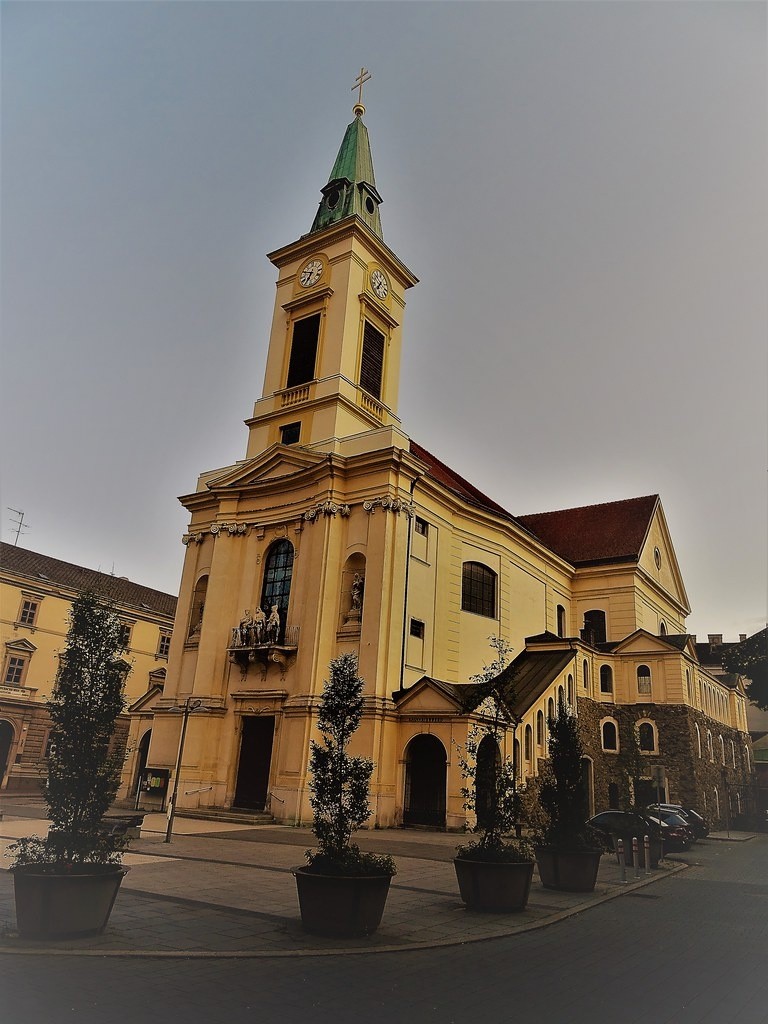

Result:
[587,809,671,860]
[642,807,698,853]
[647,801,710,844]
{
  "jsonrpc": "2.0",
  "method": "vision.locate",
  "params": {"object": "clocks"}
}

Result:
[371,268,390,301]
[299,259,324,287]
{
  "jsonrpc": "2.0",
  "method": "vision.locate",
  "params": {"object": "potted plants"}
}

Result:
[291,650,397,938]
[3,578,134,941]
[533,688,608,892]
[612,713,663,867]
[447,635,536,912]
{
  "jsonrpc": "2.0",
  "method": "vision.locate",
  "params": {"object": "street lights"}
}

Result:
[165,695,203,844]
